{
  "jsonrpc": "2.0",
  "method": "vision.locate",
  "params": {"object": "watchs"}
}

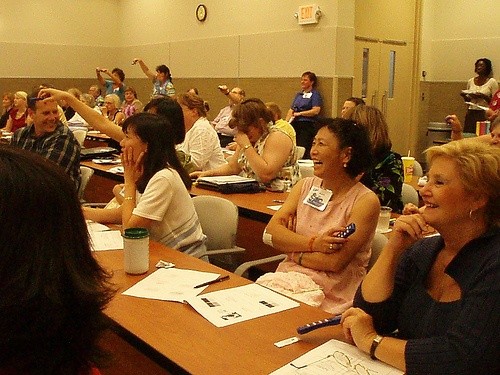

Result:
[369,334,385,360]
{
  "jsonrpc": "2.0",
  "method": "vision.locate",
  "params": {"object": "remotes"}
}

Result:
[218,86,223,89]
[337,223,356,238]
[297,317,342,334]
[30,98,44,101]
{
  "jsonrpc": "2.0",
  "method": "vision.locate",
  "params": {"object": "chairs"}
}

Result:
[192,196,245,267]
[235,233,388,314]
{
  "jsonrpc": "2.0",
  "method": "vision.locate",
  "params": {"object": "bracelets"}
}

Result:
[298,252,304,265]
[124,196,136,200]
[241,143,252,151]
[308,236,316,253]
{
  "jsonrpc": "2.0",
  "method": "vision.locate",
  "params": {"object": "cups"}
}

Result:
[401,157,414,182]
[122,228,149,274]
[377,206,392,231]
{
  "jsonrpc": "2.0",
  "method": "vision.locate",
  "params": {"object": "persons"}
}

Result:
[261,118,381,317]
[0,57,500,264]
[340,138,500,375]
[0,145,120,375]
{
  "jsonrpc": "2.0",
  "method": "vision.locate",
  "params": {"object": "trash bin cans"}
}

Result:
[426,121,453,152]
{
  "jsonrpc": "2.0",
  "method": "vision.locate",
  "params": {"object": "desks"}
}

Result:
[80,133,398,375]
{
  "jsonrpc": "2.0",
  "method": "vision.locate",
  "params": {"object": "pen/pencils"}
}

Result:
[273,199,286,203]
[193,275,230,288]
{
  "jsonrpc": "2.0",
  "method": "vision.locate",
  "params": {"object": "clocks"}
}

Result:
[196,5,207,21]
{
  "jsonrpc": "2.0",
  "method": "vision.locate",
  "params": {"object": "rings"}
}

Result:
[329,244,333,249]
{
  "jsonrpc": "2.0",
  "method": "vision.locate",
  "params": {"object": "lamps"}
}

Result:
[294,5,322,25]
